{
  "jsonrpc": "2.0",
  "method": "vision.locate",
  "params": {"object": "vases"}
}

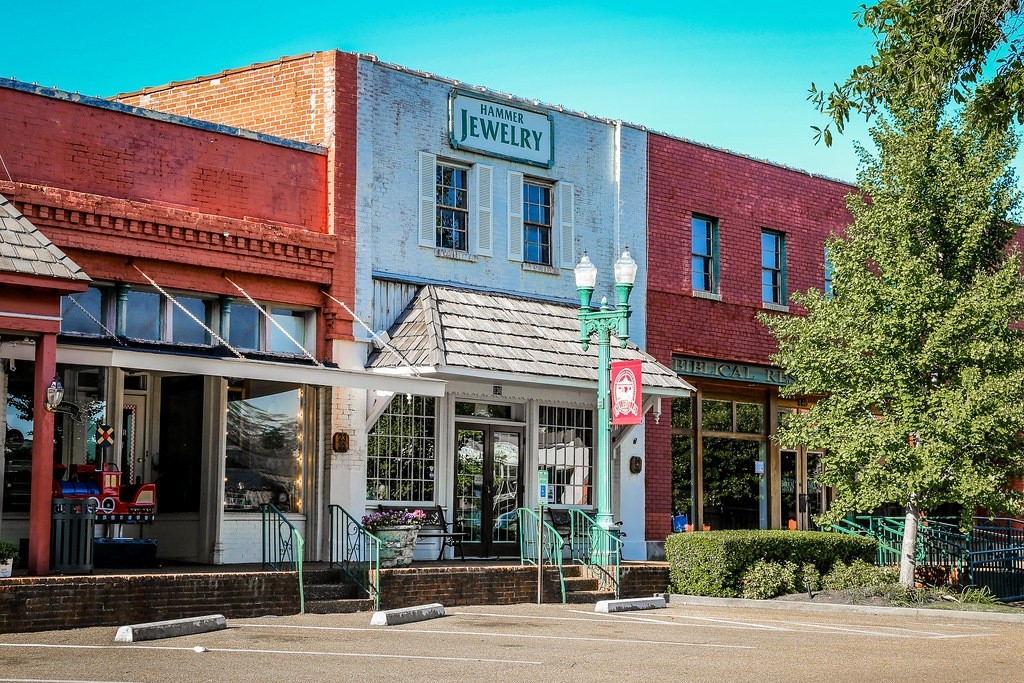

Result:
[375,526,421,568]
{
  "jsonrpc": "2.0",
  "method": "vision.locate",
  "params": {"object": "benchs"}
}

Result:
[547,507,627,561]
[378,504,467,562]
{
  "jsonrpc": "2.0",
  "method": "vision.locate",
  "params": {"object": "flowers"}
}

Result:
[360,507,426,531]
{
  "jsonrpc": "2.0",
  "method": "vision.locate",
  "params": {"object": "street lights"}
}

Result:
[572,244,639,567]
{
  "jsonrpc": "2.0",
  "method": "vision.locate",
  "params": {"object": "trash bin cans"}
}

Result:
[50,498,96,575]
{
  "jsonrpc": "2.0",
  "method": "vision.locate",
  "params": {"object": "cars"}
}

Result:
[453,505,517,540]
